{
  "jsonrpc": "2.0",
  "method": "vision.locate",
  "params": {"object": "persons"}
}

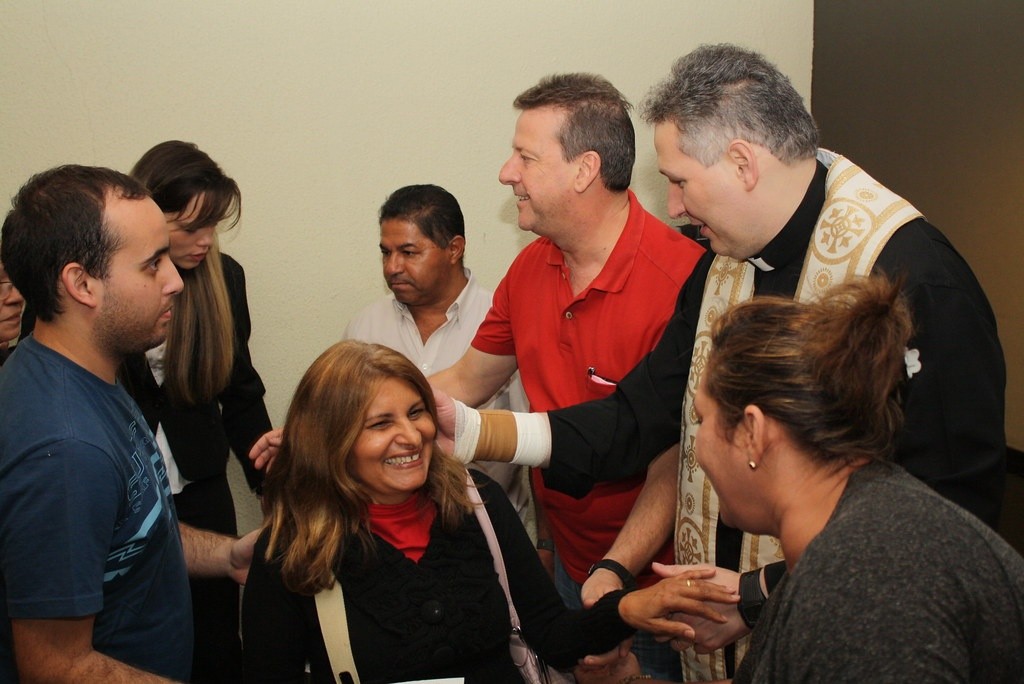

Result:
[692,279,1023,684]
[438,45,1003,684]
[241,340,739,684]
[248,72,706,684]
[340,184,529,493]
[0,139,281,684]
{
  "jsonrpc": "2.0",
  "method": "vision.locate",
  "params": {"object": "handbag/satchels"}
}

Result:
[463,469,572,684]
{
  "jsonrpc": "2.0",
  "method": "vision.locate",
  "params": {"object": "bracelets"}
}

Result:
[588,558,635,589]
[535,539,554,550]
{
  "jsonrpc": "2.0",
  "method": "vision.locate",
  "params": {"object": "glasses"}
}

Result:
[0,281,14,300]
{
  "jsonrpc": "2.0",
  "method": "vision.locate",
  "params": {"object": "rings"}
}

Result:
[686,578,691,587]
